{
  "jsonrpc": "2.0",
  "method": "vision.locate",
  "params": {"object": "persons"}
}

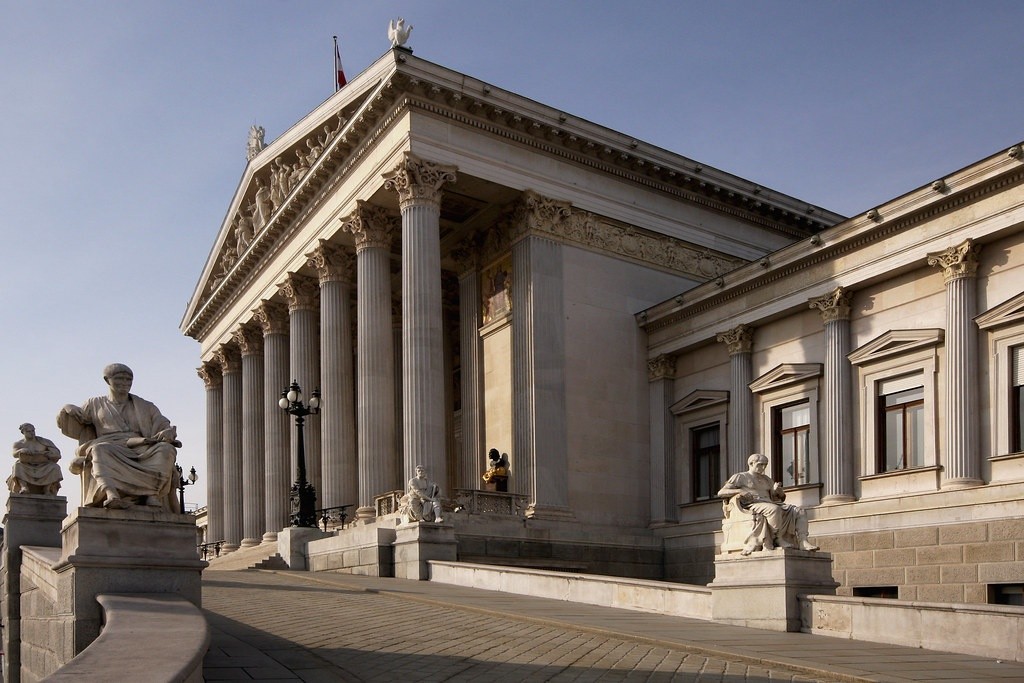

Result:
[207,111,347,290]
[401,465,445,523]
[718,454,820,555]
[58,363,177,509]
[489,448,504,468]
[12,423,63,497]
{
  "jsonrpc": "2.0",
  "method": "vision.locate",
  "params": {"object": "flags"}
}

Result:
[334,44,347,91]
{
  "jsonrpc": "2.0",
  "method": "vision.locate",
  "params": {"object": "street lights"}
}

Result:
[175,462,198,514]
[279,379,325,528]
